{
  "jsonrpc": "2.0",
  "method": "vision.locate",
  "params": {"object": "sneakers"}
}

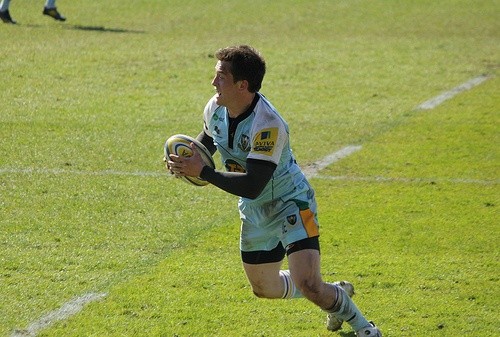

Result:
[43,6,65,20]
[327,281,354,331]
[0,10,16,24]
[355,321,382,337]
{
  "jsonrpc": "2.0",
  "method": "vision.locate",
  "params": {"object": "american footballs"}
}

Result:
[163,134,216,187]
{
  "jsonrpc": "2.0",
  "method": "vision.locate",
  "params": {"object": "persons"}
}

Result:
[0,0,67,24]
[162,44,382,337]
